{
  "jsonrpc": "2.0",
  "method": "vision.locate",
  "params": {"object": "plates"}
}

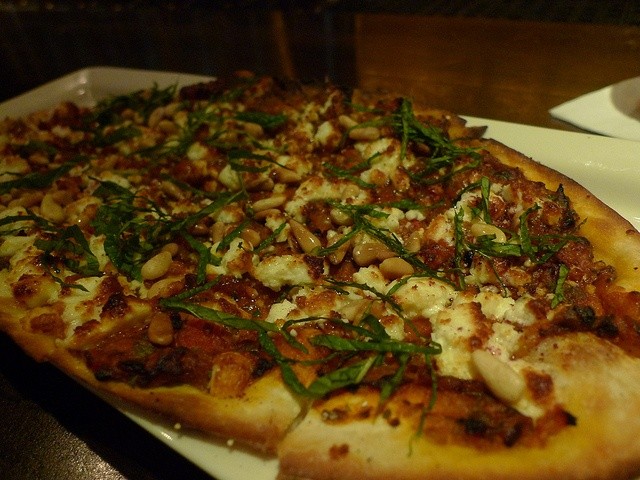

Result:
[549,73,640,139]
